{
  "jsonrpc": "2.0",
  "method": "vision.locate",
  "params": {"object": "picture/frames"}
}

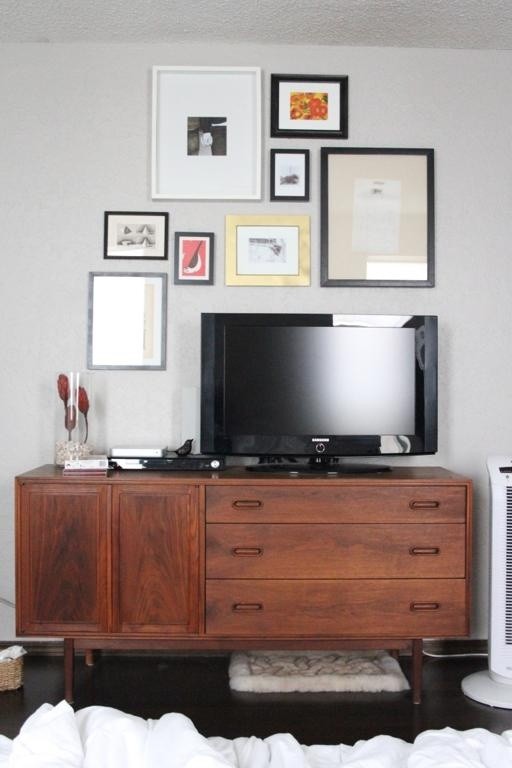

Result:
[87,266,170,373]
[147,62,265,203]
[170,230,217,289]
[266,144,312,203]
[220,211,313,287]
[267,67,352,140]
[101,208,172,262]
[317,143,437,291]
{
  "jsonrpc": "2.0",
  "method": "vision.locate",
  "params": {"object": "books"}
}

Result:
[65,454,109,469]
[63,468,107,477]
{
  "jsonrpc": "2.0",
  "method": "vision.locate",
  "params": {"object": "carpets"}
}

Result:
[230,640,413,698]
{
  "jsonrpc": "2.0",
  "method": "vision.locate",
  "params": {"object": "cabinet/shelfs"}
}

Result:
[4,458,479,712]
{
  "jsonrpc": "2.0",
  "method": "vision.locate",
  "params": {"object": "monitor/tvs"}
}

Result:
[201,313,439,474]
